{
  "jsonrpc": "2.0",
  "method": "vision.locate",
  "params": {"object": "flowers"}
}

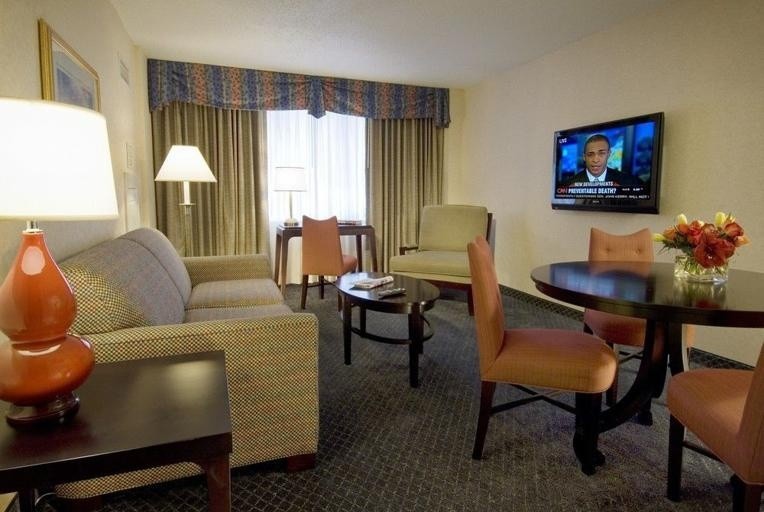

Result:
[652,210,752,277]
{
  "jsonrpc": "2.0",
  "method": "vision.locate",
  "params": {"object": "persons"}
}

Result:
[560,134,644,187]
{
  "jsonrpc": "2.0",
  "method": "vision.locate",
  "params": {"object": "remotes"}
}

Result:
[377,288,407,297]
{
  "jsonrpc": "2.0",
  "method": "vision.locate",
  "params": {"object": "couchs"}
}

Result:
[52,226,320,512]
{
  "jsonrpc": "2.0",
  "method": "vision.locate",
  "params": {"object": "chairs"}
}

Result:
[388,204,493,316]
[584,226,695,408]
[666,340,764,512]
[467,233,617,466]
[301,215,358,312]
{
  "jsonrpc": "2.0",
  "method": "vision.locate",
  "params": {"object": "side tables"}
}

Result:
[0,350,232,512]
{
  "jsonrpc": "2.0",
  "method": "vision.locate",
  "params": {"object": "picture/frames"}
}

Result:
[39,17,101,113]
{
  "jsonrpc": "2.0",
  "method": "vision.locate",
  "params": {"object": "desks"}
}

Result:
[274,224,377,300]
[531,261,764,473]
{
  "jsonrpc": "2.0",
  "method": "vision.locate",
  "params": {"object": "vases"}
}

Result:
[673,255,729,283]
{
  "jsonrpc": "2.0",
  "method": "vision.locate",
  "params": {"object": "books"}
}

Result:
[351,275,394,289]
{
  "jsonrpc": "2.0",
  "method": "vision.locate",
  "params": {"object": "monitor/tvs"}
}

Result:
[550,111,665,215]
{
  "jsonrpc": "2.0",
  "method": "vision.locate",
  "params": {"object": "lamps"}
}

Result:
[0,96,120,425]
[154,144,219,257]
[272,167,308,226]
